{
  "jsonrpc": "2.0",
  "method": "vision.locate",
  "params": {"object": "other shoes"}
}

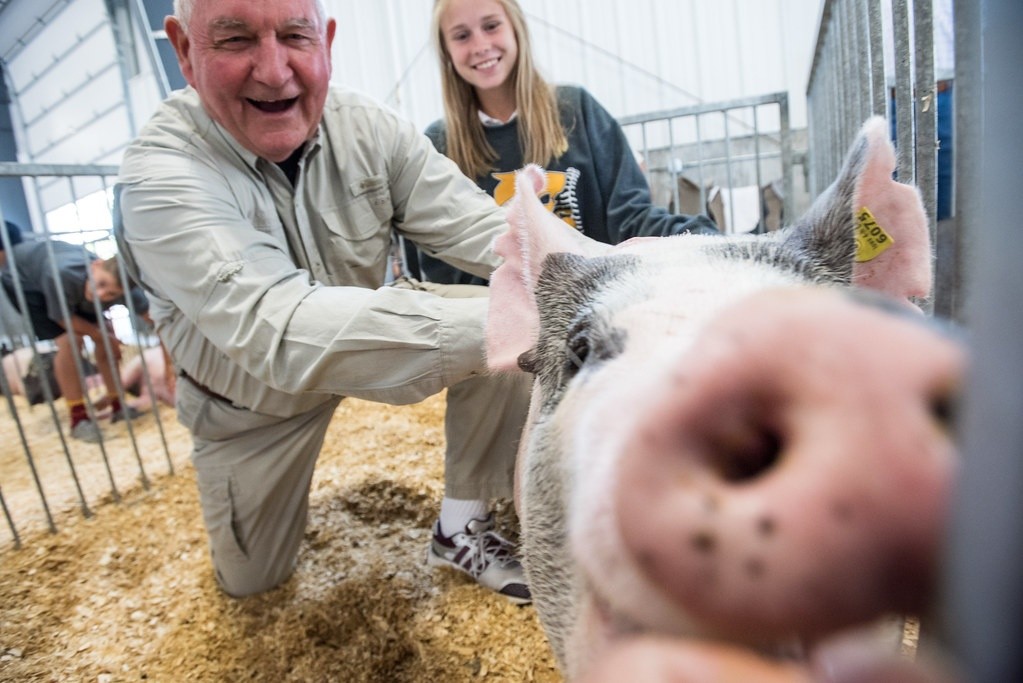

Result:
[112,406,146,422]
[69,420,99,442]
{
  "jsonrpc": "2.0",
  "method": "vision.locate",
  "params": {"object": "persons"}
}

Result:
[869,0,956,223]
[0,240,178,442]
[113,0,532,602]
[402,0,721,287]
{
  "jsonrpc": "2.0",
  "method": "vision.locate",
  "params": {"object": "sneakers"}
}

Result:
[430,510,534,607]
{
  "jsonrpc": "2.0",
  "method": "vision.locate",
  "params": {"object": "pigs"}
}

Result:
[483,116,973,683]
[100,345,176,418]
[1,343,97,407]
